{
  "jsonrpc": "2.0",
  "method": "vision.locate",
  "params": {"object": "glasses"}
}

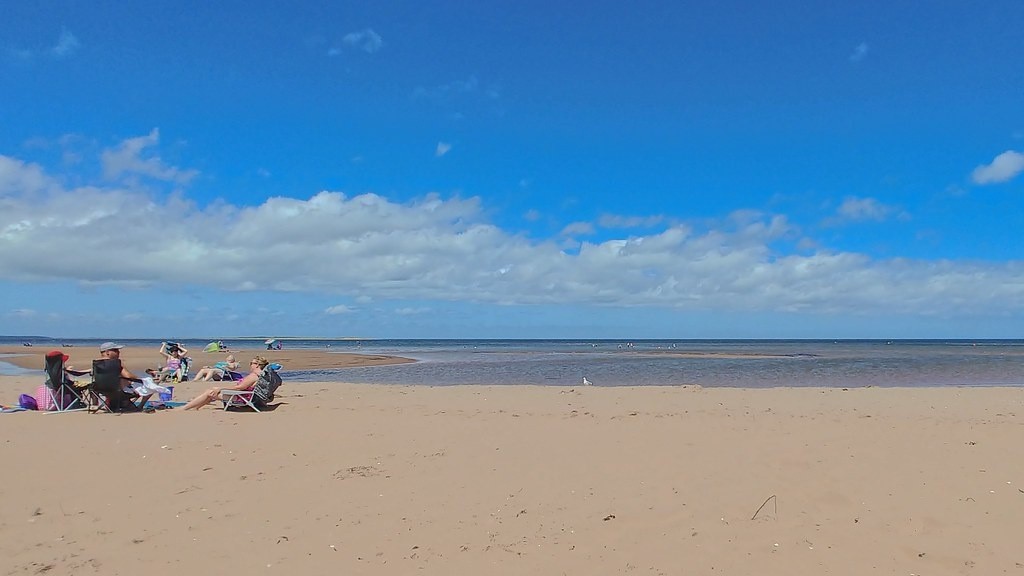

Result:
[250,362,257,365]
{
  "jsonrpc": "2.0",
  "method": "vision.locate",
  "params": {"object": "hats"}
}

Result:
[100,342,123,352]
[48,351,69,361]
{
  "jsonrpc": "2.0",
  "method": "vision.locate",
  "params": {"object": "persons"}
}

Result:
[148,342,187,384]
[24,342,32,346]
[267,344,276,350]
[219,340,226,348]
[100,342,137,409]
[325,343,361,349]
[592,342,633,349]
[48,350,84,402]
[180,356,270,411]
[277,341,282,350]
[668,343,676,349]
[193,355,236,381]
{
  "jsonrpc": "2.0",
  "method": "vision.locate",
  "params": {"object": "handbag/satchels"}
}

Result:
[35,386,71,409]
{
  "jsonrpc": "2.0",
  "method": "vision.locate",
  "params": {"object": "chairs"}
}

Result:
[86,359,143,416]
[217,360,241,382]
[43,353,91,413]
[219,362,281,413]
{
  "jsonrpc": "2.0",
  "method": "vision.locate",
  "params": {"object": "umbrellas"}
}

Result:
[265,339,275,346]
